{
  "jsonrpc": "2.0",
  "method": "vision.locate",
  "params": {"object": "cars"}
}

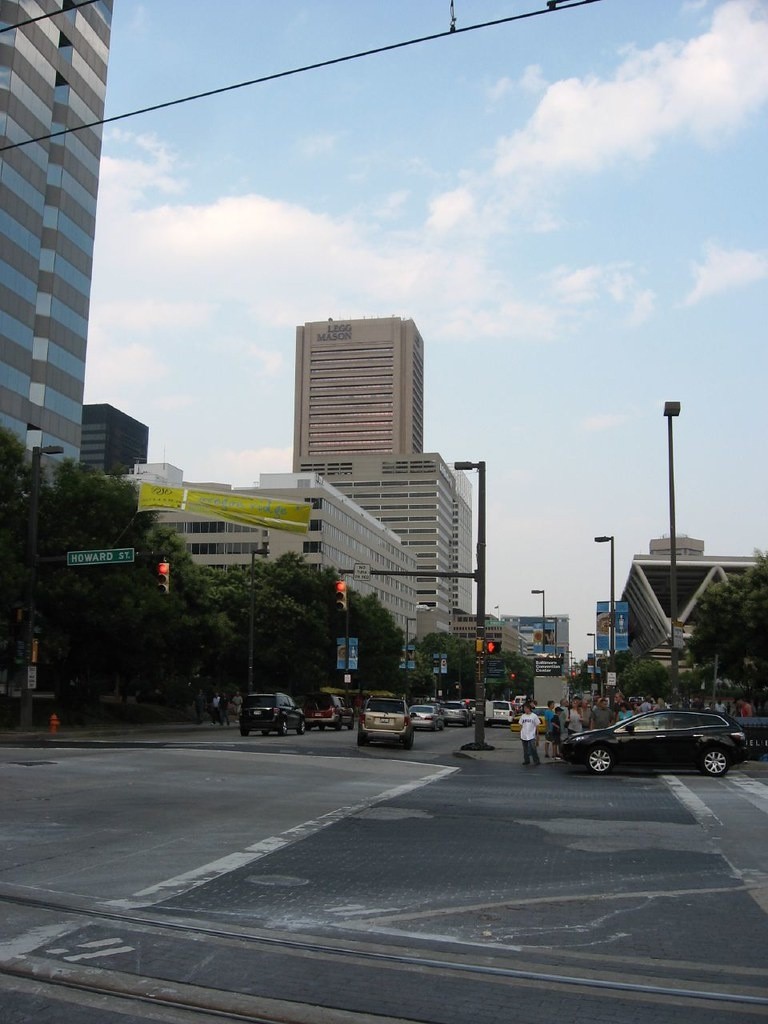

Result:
[408,704,444,731]
[462,695,555,733]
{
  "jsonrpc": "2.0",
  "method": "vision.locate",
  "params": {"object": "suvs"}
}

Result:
[441,700,474,727]
[559,709,747,777]
[237,693,354,736]
[357,696,418,748]
[486,700,515,725]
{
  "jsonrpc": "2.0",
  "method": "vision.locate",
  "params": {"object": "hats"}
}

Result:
[555,707,564,711]
[733,697,743,703]
[521,701,535,711]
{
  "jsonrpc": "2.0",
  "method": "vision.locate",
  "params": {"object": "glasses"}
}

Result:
[616,697,618,698]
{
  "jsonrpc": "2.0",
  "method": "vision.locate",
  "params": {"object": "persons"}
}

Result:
[544,692,756,762]
[519,700,541,766]
[194,691,244,727]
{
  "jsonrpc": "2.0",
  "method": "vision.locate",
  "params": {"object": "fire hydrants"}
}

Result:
[50,714,60,734]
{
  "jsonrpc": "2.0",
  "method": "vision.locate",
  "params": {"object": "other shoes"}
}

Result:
[522,762,530,765]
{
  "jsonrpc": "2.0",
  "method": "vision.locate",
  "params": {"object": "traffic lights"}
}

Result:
[591,671,594,680]
[511,673,515,680]
[488,642,501,654]
[335,580,346,612]
[157,562,170,595]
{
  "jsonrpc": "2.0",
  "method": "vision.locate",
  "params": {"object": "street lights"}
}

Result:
[456,460,485,745]
[595,536,617,721]
[532,590,546,654]
[248,549,270,693]
[664,400,682,709]
[587,632,595,686]
[18,445,63,729]
